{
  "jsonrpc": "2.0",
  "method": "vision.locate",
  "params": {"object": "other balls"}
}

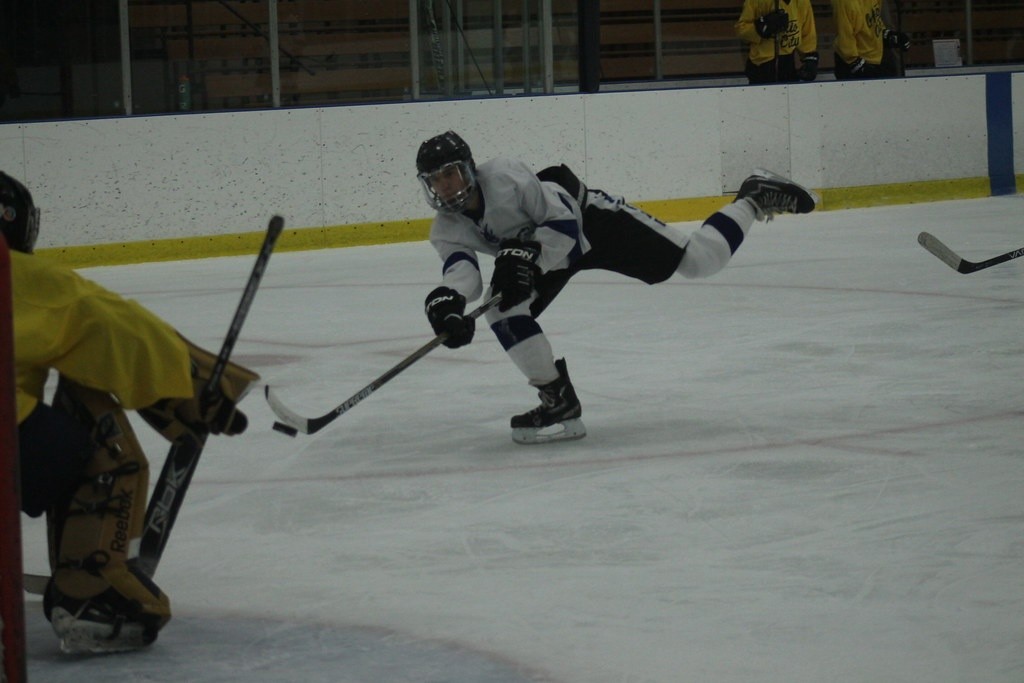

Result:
[271,420,298,438]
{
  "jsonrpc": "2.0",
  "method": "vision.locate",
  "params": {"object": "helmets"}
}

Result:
[416,131,478,216]
[0,171,40,253]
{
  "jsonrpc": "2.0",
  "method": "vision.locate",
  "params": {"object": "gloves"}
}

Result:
[883,29,912,51]
[848,58,872,78]
[796,52,819,81]
[425,286,476,349]
[754,9,789,40]
[490,238,541,312]
[169,376,234,435]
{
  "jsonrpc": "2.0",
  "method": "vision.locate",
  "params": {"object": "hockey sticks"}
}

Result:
[263,291,502,436]
[13,214,287,598]
[895,0,908,80]
[769,0,785,85]
[917,230,1024,276]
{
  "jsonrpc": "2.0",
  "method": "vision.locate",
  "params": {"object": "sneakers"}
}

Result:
[732,168,821,224]
[511,357,587,444]
[43,576,160,653]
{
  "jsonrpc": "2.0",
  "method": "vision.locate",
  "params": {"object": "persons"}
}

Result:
[416,130,815,429]
[0,171,261,650]
[831,0,910,80]
[734,0,820,85]
